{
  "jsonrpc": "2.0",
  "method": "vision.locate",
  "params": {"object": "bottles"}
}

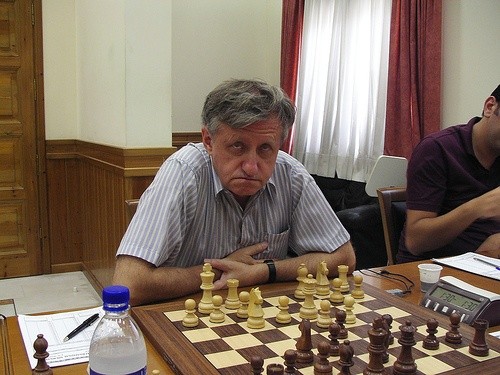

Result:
[90,286,148,375]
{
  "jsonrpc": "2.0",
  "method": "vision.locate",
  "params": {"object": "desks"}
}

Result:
[1,255,500,375]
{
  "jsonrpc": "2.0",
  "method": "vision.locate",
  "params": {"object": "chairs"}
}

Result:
[376,185,407,266]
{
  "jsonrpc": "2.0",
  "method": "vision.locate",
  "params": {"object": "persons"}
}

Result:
[397,84,500,265]
[113,80,355,307]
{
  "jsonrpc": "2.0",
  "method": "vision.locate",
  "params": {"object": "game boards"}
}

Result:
[130,276,500,375]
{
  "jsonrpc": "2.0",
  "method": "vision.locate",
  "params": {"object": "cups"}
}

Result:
[418,264,443,293]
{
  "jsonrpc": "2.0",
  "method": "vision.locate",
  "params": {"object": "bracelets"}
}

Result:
[263,260,276,283]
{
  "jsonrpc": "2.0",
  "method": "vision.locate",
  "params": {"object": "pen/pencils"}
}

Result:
[63,313,99,342]
[474,258,500,271]
